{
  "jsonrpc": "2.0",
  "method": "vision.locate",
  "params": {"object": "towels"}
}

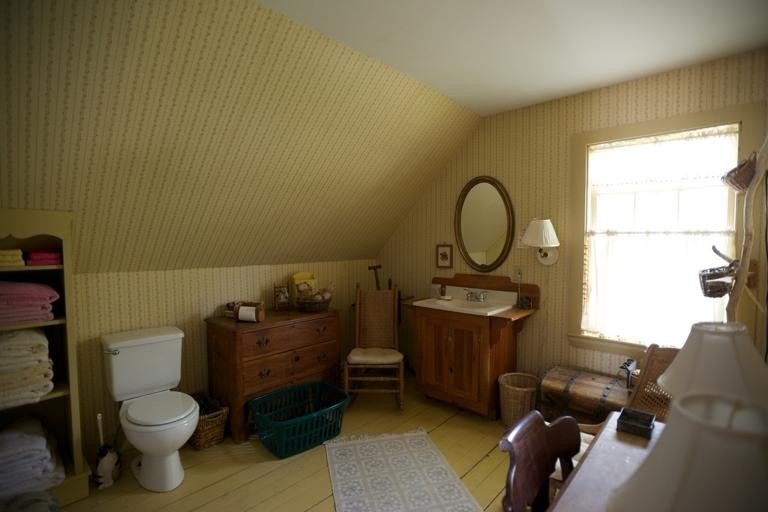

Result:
[1,247,69,499]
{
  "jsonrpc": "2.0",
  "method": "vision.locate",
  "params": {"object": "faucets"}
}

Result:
[464,288,488,302]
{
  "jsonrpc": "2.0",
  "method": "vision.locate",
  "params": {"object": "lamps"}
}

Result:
[656,319,767,408]
[520,218,561,267]
[603,393,768,511]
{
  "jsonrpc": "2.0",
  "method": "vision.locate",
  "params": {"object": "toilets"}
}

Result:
[98,324,201,493]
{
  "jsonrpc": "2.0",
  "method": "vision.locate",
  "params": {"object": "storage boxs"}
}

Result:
[541,368,629,425]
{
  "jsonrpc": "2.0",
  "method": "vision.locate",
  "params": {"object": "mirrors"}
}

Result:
[454,175,516,273]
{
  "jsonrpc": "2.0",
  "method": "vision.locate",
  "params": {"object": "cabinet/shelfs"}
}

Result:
[0,207,92,512]
[206,305,346,444]
[414,308,520,421]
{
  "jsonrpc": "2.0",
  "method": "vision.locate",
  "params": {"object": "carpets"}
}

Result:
[321,427,484,511]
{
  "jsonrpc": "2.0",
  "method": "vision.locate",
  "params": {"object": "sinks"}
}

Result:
[412,285,517,316]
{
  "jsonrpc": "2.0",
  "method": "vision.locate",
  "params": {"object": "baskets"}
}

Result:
[295,296,333,312]
[497,372,542,430]
[186,391,230,451]
[249,379,351,461]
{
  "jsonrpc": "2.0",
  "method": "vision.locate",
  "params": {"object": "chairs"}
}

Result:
[549,336,682,505]
[342,286,409,409]
[498,408,583,511]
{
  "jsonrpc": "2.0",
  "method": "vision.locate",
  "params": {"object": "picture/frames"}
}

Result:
[436,245,454,269]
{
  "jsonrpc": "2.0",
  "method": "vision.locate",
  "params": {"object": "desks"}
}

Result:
[545,410,668,511]
